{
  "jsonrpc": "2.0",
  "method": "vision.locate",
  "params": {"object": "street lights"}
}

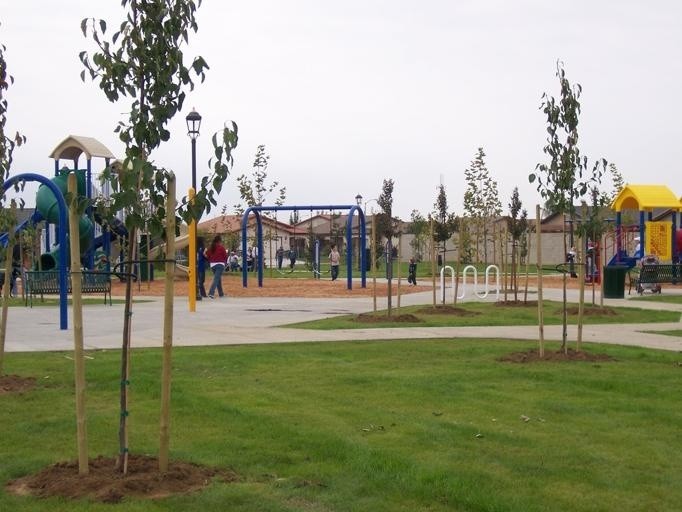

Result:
[185,106,204,301]
[364,198,379,220]
[356,193,362,270]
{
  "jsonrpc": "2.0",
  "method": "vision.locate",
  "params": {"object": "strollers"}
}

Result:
[635,254,662,294]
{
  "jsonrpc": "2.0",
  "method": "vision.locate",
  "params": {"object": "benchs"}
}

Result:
[628,264,682,297]
[25,270,113,308]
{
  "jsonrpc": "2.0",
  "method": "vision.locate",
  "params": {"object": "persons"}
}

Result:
[225,242,259,273]
[12,234,34,298]
[327,243,340,281]
[274,245,283,268]
[633,237,640,252]
[207,235,228,299]
[196,239,209,301]
[407,257,417,286]
[286,245,298,268]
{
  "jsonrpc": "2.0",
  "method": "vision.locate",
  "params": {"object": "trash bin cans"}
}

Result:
[603,265,630,298]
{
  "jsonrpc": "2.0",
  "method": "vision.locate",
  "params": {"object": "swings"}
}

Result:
[274,209,298,275]
[310,209,333,275]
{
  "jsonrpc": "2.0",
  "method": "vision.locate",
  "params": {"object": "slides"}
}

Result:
[148,220,190,279]
[0,172,129,283]
